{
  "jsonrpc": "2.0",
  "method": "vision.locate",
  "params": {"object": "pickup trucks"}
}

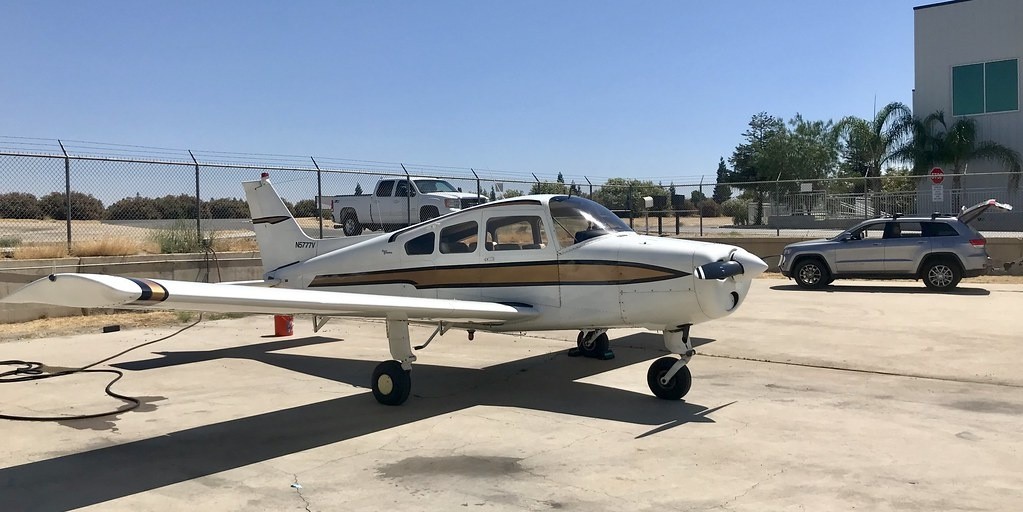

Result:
[331,174,488,237]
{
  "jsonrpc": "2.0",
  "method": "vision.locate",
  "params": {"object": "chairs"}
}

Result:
[883,223,901,238]
[440,240,546,254]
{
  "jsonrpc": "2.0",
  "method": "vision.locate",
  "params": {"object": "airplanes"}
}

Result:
[0,173,769,410]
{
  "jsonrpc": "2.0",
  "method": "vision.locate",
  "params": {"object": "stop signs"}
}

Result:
[930,167,944,184]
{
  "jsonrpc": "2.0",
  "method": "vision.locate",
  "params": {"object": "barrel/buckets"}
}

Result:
[274,314,293,336]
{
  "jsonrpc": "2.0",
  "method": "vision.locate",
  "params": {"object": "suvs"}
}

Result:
[776,198,1012,294]
